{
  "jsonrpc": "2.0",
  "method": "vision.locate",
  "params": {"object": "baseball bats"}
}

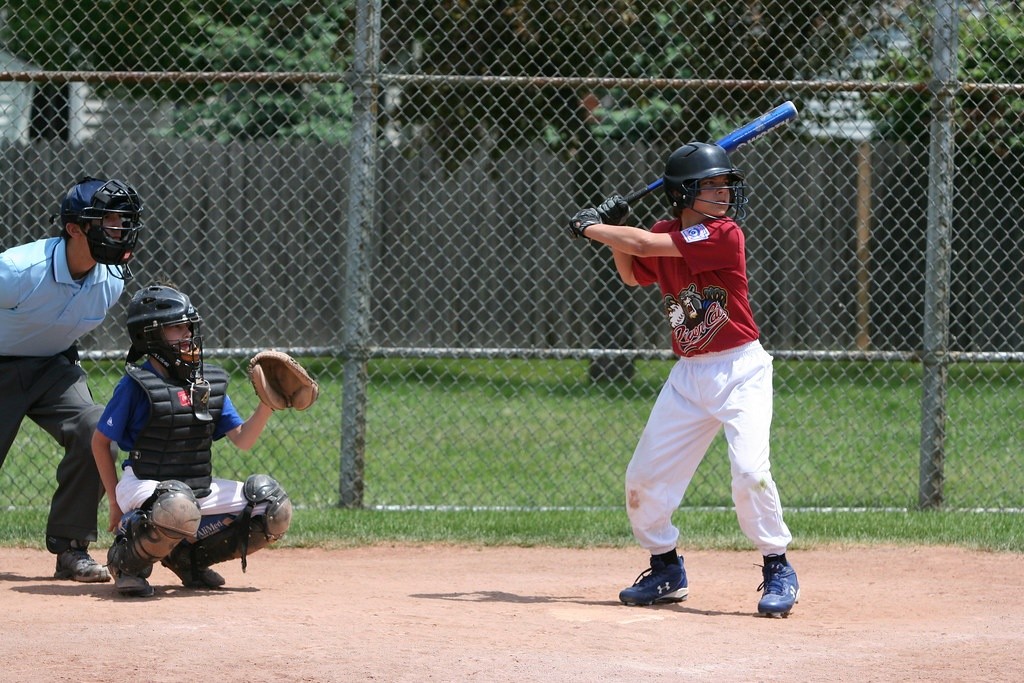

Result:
[571,101,800,232]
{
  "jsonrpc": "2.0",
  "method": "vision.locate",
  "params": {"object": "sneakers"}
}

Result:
[754,554,798,617]
[160,553,207,586]
[619,556,688,606]
[107,560,154,597]
[54,539,111,582]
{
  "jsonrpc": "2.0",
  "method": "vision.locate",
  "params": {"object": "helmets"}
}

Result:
[61,179,130,224]
[126,286,203,377]
[663,142,748,221]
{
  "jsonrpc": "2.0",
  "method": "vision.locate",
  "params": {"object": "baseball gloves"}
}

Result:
[247,349,320,411]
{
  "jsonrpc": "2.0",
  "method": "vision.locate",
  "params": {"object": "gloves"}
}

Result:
[596,195,631,226]
[569,208,602,239]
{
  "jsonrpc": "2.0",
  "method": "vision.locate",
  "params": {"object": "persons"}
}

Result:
[571,142,800,618]
[0,177,143,580]
[91,286,319,596]
